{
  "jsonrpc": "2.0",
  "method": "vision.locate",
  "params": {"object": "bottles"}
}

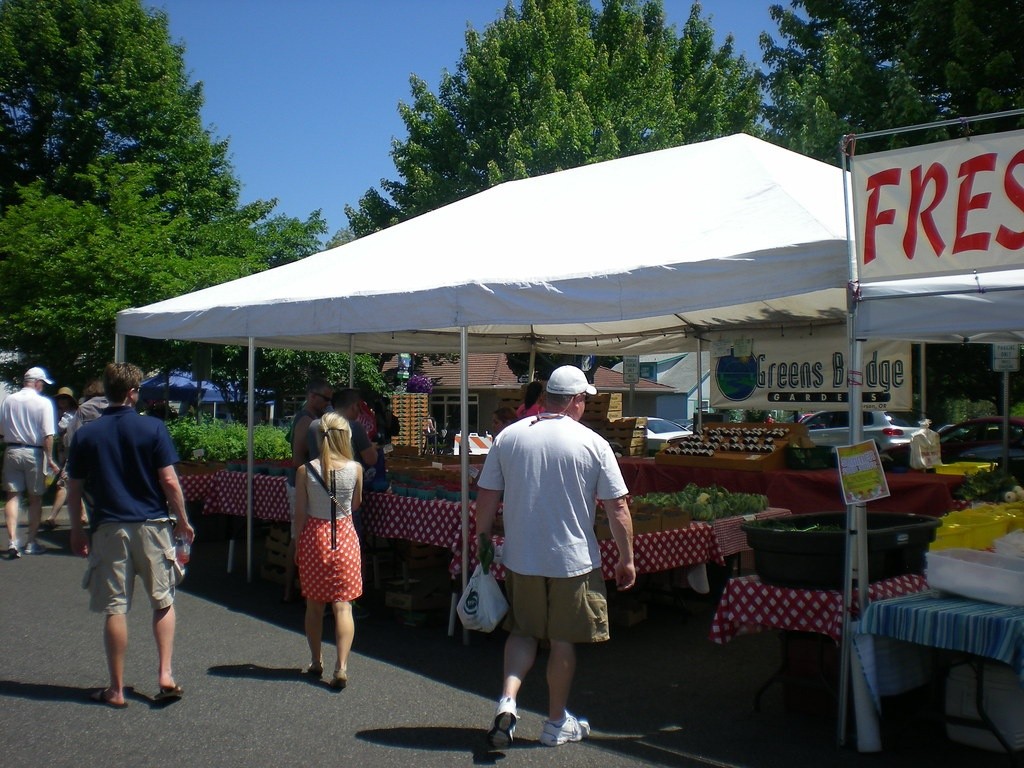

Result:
[665,426,790,457]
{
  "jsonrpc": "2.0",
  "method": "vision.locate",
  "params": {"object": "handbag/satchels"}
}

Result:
[354,447,391,494]
[455,562,509,633]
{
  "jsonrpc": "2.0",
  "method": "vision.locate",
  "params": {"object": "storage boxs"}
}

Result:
[935,461,997,477]
[924,549,1024,605]
[392,393,430,448]
[258,525,290,581]
[385,588,433,610]
[927,498,1024,550]
[493,387,648,455]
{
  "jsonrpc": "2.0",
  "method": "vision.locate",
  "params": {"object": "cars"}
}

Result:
[801,410,922,457]
[623,415,694,454]
[786,413,833,429]
[935,414,1024,471]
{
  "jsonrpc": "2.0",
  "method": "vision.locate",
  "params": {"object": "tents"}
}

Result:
[115,131,1024,756]
[137,368,276,426]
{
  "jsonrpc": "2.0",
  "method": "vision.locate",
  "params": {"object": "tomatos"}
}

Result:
[392,477,479,491]
[228,459,293,467]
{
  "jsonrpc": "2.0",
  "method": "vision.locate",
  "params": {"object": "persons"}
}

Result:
[283,381,399,610]
[291,412,362,687]
[0,367,60,557]
[41,380,109,528]
[515,381,545,419]
[64,363,194,708]
[492,406,517,435]
[474,366,637,747]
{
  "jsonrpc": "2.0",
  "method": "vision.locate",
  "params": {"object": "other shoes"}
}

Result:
[44,519,64,530]
[308,662,325,676]
[329,672,350,688]
[81,518,91,526]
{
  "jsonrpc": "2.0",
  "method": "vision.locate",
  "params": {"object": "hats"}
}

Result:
[546,364,602,394]
[53,386,77,402]
[23,367,55,386]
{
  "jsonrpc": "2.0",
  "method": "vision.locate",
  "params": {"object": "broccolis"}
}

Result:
[636,482,769,520]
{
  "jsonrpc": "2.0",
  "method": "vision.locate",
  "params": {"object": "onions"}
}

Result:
[1005,486,1024,502]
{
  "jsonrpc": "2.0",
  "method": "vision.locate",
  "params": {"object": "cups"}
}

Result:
[176,531,190,563]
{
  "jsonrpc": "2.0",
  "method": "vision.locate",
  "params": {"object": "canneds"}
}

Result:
[665,427,790,456]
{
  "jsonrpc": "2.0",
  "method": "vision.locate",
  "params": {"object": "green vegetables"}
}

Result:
[747,519,843,532]
[953,463,1015,502]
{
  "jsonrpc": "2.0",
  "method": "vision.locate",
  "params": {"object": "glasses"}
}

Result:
[314,392,331,402]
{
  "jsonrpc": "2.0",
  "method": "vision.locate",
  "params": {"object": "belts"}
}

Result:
[7,442,44,448]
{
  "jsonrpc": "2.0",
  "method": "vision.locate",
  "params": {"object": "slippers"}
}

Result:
[92,689,130,709]
[153,685,184,700]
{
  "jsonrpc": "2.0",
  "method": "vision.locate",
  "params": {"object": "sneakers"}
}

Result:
[8,541,22,559]
[484,697,523,747]
[538,712,597,746]
[24,541,55,555]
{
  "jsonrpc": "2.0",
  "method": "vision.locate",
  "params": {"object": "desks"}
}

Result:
[706,575,922,715]
[860,596,1024,758]
[175,473,786,646]
